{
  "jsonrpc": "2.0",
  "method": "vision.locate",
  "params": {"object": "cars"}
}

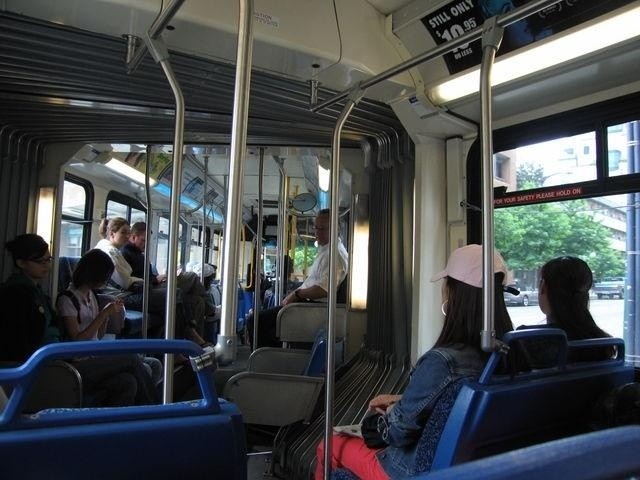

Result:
[505,288,539,306]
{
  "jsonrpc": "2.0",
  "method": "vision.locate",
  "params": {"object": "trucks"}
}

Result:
[594,276,624,299]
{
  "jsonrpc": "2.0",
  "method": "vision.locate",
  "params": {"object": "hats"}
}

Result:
[430,244,507,289]
[193,263,214,278]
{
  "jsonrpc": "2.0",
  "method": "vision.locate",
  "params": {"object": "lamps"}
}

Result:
[96,153,222,221]
[416,0,640,112]
[318,157,330,192]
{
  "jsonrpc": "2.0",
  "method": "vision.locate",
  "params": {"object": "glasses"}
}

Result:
[24,257,55,265]
[313,225,323,230]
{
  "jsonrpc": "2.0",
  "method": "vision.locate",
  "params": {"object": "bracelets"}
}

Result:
[293,287,302,302]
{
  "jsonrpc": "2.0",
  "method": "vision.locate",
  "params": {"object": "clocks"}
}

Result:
[293,193,317,212]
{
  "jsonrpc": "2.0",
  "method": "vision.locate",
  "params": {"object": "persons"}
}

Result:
[263,255,305,309]
[192,262,221,344]
[0,232,163,407]
[246,208,349,353]
[515,255,618,363]
[120,221,221,324]
[93,216,215,365]
[313,242,534,480]
[55,248,163,383]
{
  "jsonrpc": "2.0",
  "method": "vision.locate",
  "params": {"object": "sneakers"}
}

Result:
[203,344,216,353]
